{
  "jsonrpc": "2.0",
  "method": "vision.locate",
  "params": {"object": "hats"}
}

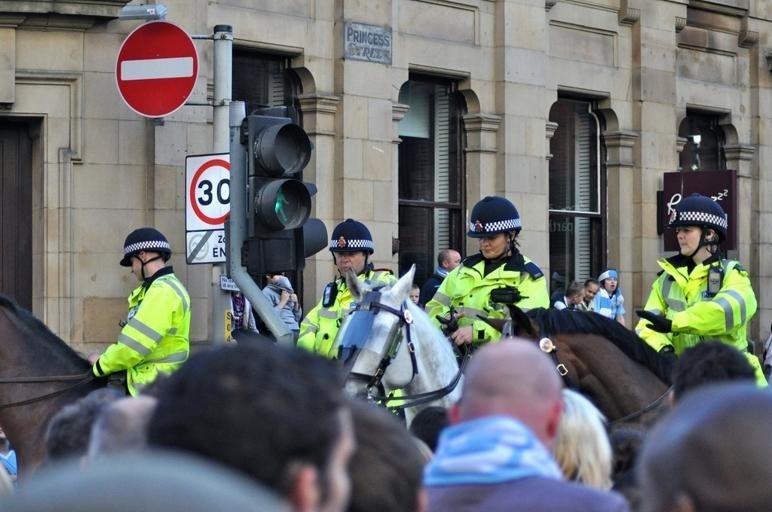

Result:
[596,268,619,282]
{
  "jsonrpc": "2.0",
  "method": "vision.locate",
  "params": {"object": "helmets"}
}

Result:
[666,191,730,242]
[118,226,173,267]
[326,217,376,255]
[465,194,523,239]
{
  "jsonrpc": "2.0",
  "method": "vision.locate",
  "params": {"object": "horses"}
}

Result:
[0,292,126,493]
[333,261,466,434]
[475,303,675,428]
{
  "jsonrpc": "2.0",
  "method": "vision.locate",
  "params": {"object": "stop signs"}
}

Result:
[115,20,199,118]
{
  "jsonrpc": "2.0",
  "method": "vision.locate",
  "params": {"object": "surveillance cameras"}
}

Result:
[687,135,701,146]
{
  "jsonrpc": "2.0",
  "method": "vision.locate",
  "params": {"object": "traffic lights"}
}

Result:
[295,179,329,265]
[244,112,315,279]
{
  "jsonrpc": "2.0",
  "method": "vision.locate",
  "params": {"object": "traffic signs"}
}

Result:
[184,153,236,234]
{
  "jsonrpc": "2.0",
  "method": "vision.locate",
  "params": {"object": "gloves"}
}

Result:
[634,307,673,334]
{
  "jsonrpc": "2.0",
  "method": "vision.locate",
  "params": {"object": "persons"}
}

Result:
[552,280,586,314]
[261,272,303,344]
[633,192,770,389]
[296,218,406,421]
[421,248,462,306]
[423,195,551,377]
[231,289,261,337]
[591,267,626,327]
[87,227,191,397]
[577,279,600,314]
[408,283,420,305]
